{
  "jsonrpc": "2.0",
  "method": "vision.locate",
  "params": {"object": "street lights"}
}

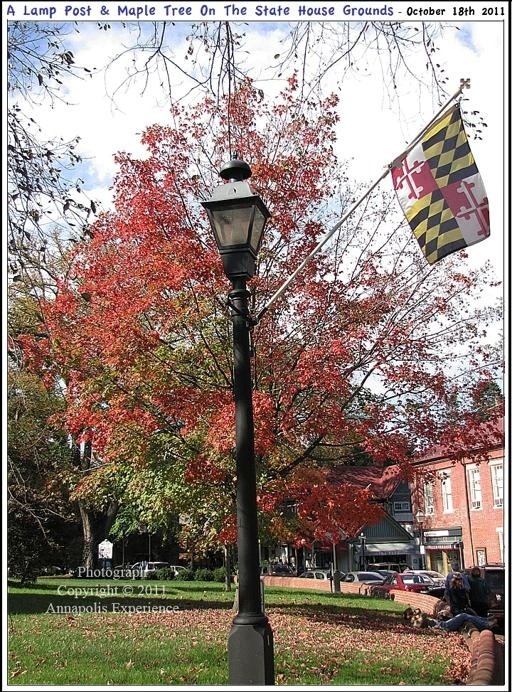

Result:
[200,149,276,685]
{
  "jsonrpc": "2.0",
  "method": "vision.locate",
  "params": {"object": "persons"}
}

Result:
[445,562,492,615]
[427,613,502,637]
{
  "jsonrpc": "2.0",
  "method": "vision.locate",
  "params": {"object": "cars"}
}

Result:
[261,563,505,623]
[113,561,188,579]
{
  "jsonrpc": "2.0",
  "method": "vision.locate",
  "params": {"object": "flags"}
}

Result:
[390,102,490,266]
